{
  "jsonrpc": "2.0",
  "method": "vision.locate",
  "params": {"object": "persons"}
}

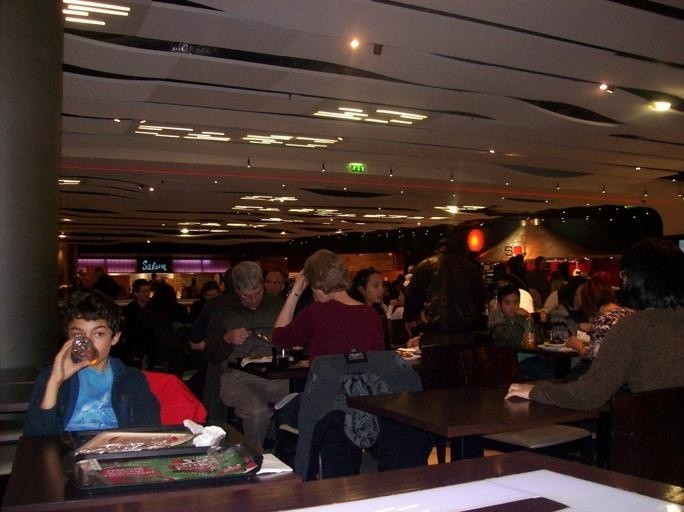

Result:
[272,249,384,451]
[503,240,684,411]
[119,273,199,378]
[349,239,484,388]
[487,255,634,379]
[92,267,120,299]
[188,259,289,451]
[24,289,160,437]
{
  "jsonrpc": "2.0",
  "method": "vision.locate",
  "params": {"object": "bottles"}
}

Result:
[277,345,289,371]
[72,337,99,366]
[524,313,535,349]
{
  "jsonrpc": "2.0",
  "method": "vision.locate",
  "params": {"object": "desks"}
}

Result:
[0,336,684,512]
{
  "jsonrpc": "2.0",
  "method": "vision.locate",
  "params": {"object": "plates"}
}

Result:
[399,354,421,360]
[542,341,567,347]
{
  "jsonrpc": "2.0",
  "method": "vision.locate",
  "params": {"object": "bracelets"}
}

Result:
[290,292,299,297]
[576,345,584,351]
